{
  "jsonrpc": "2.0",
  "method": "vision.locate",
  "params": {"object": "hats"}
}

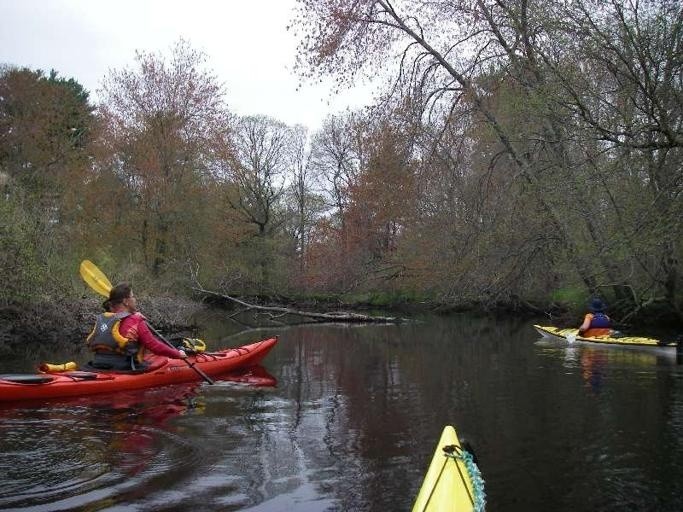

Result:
[588,299,607,311]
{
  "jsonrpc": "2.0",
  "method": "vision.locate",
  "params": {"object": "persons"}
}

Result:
[578,297,612,337]
[85,284,187,373]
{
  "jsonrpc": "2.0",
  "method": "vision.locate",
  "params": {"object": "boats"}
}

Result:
[409,423,489,511]
[43,363,280,421]
[528,321,676,351]
[534,339,659,363]
[0,332,281,402]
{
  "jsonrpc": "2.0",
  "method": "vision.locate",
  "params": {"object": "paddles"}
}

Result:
[80,260,214,384]
[567,330,579,343]
